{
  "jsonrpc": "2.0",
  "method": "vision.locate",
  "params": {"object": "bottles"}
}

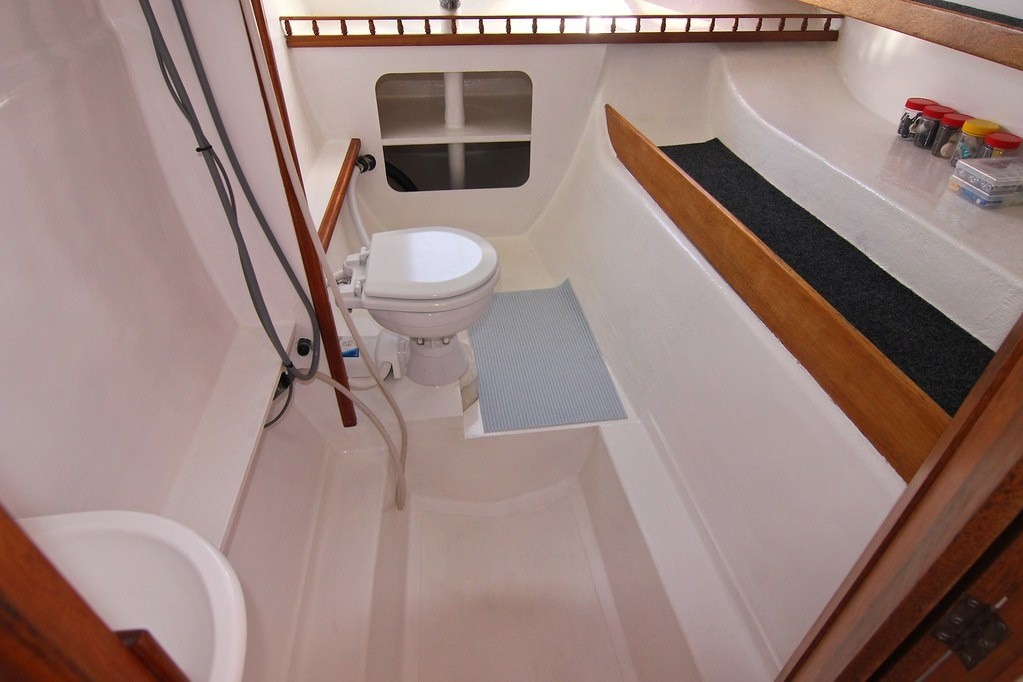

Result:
[931,114,976,158]
[951,118,999,169]
[897,98,937,142]
[912,105,955,150]
[978,131,1022,159]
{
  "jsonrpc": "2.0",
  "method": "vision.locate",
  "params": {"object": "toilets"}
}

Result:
[334,225,501,388]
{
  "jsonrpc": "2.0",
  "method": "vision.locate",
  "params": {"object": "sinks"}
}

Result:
[12,509,249,682]
